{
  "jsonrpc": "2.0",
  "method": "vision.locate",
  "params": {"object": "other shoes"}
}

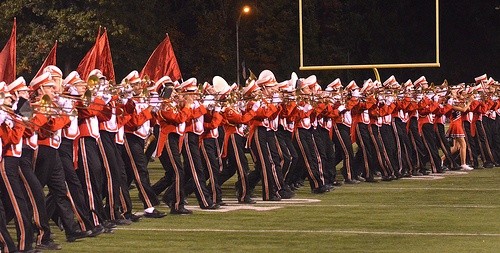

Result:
[233,158,498,204]
[87,212,143,236]
[144,208,168,218]
[170,208,192,214]
[35,241,63,250]
[205,202,220,208]
[66,229,92,241]
[26,247,41,252]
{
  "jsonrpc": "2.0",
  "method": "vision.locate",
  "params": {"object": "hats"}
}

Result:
[203,69,500,93]
[155,75,174,93]
[1,64,107,99]
[122,69,142,86]
[181,77,199,92]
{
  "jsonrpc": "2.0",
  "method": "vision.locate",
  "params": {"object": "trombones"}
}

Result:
[0,70,496,143]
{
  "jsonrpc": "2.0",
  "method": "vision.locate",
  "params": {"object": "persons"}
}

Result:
[0,64,500,253]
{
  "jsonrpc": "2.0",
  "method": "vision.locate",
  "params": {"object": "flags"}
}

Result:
[32,42,57,80]
[76,26,117,86]
[139,35,183,83]
[0,17,17,86]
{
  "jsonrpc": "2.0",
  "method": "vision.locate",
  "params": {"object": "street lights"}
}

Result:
[236,5,251,86]
